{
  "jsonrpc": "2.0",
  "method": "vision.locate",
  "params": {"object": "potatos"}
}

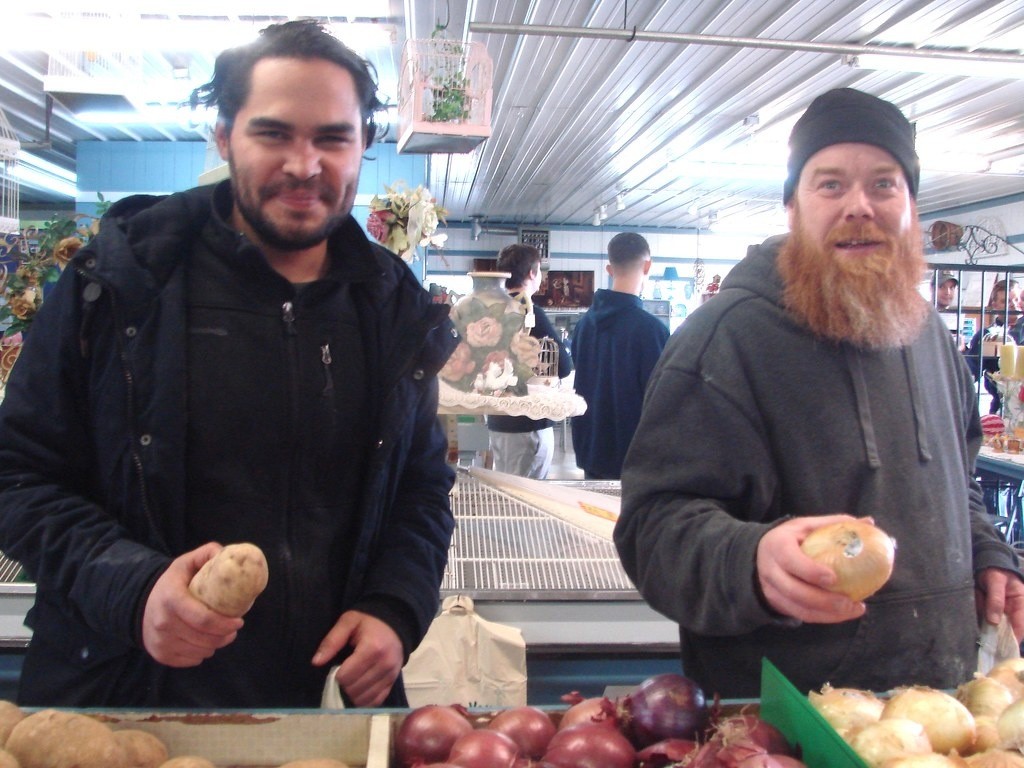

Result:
[188,543,268,619]
[0,697,350,768]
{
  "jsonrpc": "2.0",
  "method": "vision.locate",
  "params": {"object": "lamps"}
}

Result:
[599,205,608,220]
[839,53,1024,79]
[592,213,601,227]
[687,199,701,216]
[616,195,626,211]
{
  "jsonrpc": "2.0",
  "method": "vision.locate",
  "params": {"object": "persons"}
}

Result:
[1,20,465,707]
[929,270,963,345]
[953,280,1024,413]
[612,88,1024,700]
[563,330,574,369]
[571,232,671,480]
[484,244,572,479]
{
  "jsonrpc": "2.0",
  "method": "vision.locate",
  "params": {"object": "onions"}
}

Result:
[395,673,809,768]
[805,655,1024,768]
[800,520,894,603]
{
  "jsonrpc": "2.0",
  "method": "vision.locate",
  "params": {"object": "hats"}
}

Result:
[932,267,959,288]
[783,88,921,206]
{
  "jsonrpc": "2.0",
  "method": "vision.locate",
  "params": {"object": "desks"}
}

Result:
[974,446,1024,549]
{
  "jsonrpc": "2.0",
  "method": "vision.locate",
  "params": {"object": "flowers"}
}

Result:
[364,178,453,267]
[0,189,110,387]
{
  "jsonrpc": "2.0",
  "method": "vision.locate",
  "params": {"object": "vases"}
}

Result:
[440,271,541,395]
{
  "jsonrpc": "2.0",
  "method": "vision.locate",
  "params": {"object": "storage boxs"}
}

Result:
[7,688,956,768]
